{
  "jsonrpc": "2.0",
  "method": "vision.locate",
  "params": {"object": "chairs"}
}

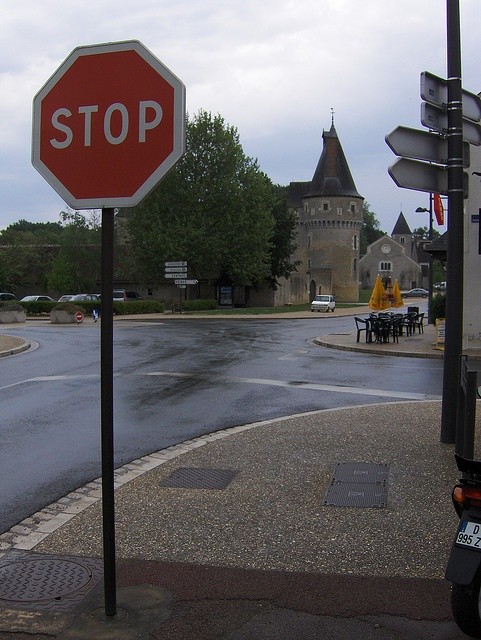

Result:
[413,313,425,334]
[369,312,417,344]
[354,317,369,343]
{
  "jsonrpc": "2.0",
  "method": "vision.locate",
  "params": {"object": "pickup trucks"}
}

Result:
[310,295,335,312]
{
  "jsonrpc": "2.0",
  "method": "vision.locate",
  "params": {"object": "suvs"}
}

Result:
[112,289,142,301]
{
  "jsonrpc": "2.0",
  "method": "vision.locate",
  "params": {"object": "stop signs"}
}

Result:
[30,39,184,209]
[75,313,82,322]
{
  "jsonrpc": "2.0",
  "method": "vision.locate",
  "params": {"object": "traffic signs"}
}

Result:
[164,274,186,278]
[165,268,186,272]
[164,262,186,266]
[174,280,197,284]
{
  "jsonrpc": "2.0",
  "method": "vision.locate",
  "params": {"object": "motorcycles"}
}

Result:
[445,453,480,633]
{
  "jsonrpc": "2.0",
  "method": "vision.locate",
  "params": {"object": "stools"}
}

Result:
[285,303,293,307]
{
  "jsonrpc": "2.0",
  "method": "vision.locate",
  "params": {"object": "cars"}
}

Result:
[19,295,54,301]
[57,295,73,301]
[68,294,99,301]
[0,293,17,301]
[400,287,429,298]
[433,280,446,291]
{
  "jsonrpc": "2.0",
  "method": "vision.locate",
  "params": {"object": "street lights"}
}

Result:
[415,193,437,325]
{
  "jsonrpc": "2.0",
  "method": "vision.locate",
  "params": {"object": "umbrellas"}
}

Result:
[368,273,391,316]
[384,275,395,313]
[392,279,405,314]
